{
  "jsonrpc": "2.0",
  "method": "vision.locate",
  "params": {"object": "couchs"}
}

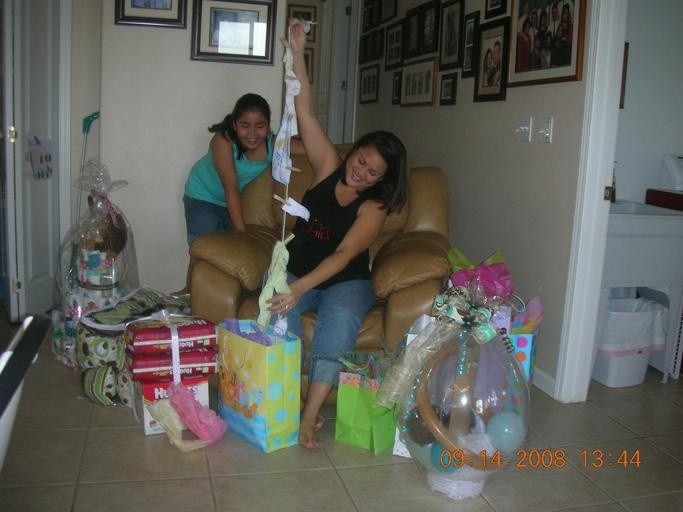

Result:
[187,144,452,407]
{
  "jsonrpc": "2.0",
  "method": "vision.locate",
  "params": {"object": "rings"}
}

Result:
[284,304,290,310]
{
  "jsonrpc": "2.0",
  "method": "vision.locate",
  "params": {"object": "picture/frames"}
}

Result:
[191,0,277,66]
[302,49,314,84]
[359,0,587,108]
[114,1,188,29]
[286,4,316,43]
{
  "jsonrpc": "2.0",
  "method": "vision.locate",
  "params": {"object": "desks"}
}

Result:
[589,202,683,384]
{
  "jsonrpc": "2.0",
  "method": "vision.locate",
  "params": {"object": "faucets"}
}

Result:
[613,158,619,203]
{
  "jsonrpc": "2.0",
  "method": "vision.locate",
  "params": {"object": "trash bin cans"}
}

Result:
[592,298,669,388]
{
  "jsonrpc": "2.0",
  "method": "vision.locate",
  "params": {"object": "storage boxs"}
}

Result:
[129,376,211,436]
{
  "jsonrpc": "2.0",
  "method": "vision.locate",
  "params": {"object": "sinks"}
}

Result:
[603,202,682,289]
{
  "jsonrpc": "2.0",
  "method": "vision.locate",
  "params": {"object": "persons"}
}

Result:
[258,17,410,452]
[359,0,577,105]
[166,91,348,301]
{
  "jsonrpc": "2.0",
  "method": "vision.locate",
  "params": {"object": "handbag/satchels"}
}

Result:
[335,313,437,460]
[219,319,300,454]
